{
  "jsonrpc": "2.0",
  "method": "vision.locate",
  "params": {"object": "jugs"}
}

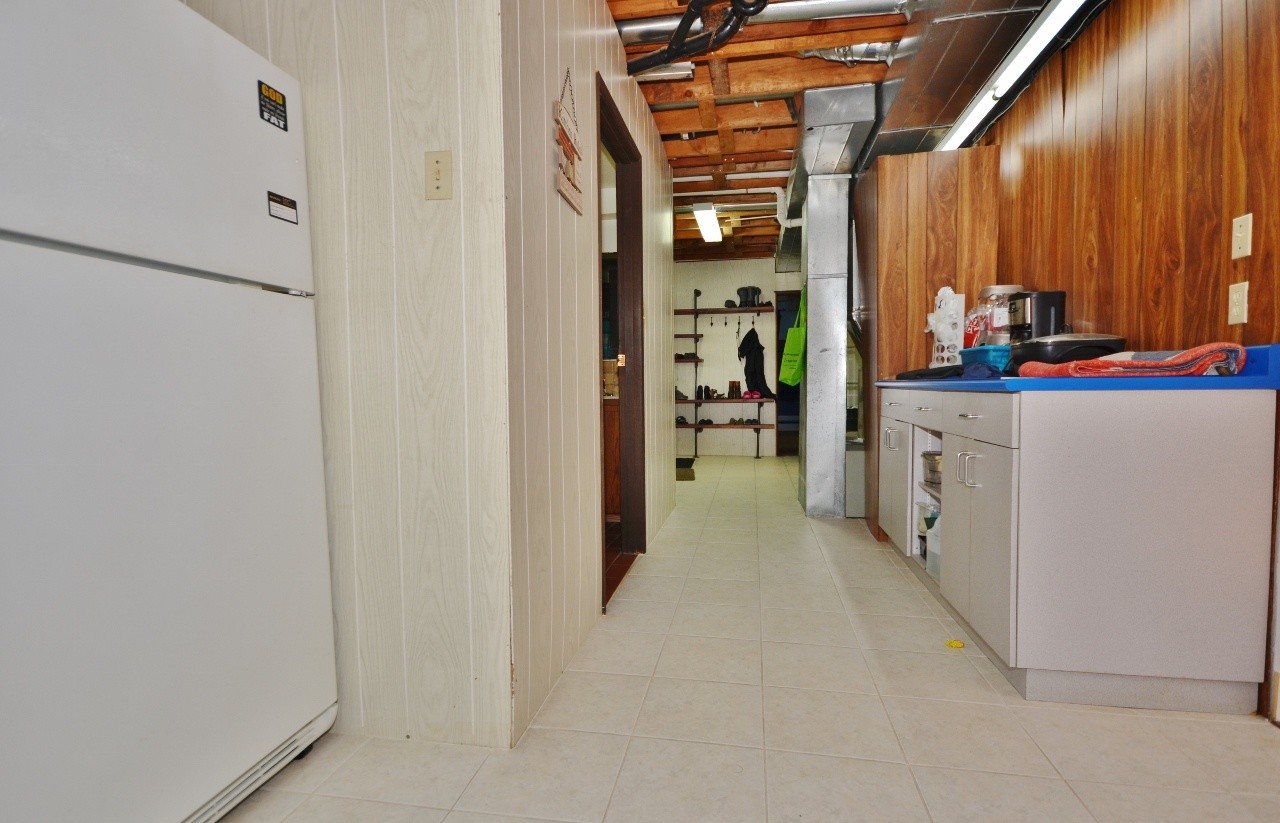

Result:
[1009,330,1031,343]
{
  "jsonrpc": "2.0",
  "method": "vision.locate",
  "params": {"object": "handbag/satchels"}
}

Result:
[779,286,807,387]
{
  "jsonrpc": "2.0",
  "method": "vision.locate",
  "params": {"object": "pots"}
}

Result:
[1011,323,1126,368]
[921,450,941,484]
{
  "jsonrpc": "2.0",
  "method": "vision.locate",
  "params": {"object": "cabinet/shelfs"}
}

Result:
[602,399,620,521]
[855,144,1275,715]
[673,290,776,456]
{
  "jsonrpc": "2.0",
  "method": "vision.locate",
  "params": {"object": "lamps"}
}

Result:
[693,202,723,242]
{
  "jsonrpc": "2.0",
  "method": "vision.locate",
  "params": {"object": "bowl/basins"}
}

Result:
[914,501,941,533]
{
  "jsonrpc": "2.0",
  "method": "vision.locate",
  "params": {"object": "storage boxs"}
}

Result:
[926,530,942,579]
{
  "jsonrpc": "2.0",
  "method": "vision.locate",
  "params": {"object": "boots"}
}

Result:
[697,385,703,400]
[737,287,747,307]
[747,286,756,307]
[734,381,741,399]
[729,381,734,399]
[704,386,710,400]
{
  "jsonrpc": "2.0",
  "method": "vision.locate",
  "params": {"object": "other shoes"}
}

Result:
[743,391,752,399]
[751,419,758,424]
[752,391,760,399]
[737,418,744,424]
[684,353,696,359]
[706,419,713,425]
[745,419,751,425]
[675,390,688,400]
[675,354,684,359]
[699,419,706,424]
[676,416,688,424]
[730,418,737,424]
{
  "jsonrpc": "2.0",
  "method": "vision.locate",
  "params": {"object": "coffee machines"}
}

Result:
[1006,290,1066,375]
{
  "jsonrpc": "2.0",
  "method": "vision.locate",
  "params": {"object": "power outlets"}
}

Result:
[1228,281,1248,325]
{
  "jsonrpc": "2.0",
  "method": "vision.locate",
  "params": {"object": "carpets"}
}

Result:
[676,468,695,481]
[675,458,695,468]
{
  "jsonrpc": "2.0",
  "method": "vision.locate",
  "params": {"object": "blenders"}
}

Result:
[975,284,1023,345]
